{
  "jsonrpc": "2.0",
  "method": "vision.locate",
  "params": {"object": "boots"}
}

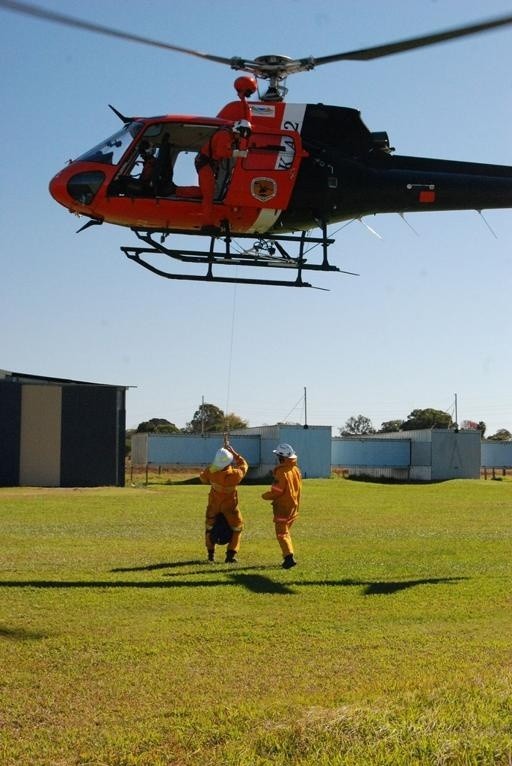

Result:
[208,549,214,561]
[224,550,237,563]
[282,554,296,569]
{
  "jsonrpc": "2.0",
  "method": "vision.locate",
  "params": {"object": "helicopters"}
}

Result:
[0,0,512,293]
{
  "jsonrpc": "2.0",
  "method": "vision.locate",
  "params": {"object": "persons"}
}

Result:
[139,140,158,187]
[261,441,303,569]
[198,432,249,563]
[195,119,252,233]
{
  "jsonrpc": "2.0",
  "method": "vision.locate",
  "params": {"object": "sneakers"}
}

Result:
[200,224,222,233]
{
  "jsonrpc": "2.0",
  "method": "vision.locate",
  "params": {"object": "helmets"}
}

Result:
[233,119,251,136]
[214,448,233,470]
[273,443,294,457]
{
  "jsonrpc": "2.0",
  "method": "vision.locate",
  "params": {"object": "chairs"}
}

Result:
[119,156,157,188]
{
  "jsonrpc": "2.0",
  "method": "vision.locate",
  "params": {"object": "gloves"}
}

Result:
[232,150,248,159]
